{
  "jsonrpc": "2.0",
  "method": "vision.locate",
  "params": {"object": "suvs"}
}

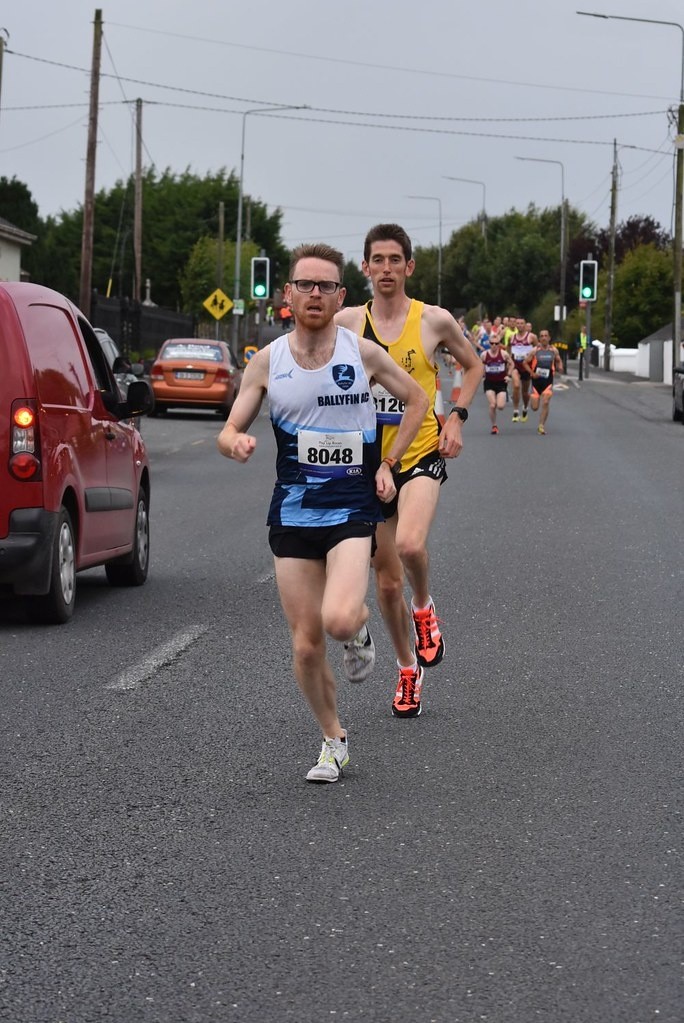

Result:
[91,327,144,437]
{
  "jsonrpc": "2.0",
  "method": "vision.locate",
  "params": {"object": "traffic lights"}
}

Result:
[251,256,269,299]
[580,260,597,302]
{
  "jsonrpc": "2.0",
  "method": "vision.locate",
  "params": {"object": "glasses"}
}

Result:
[489,341,500,346]
[288,279,342,294]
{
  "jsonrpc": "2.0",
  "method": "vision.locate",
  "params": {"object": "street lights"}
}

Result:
[407,195,442,308]
[516,158,564,340]
[441,177,485,235]
[233,105,310,355]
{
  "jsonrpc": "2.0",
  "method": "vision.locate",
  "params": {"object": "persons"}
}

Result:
[217,243,429,781]
[442,313,592,436]
[333,225,484,714]
[264,304,295,332]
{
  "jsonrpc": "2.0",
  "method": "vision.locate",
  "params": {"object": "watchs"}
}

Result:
[448,407,468,423]
[382,458,403,475]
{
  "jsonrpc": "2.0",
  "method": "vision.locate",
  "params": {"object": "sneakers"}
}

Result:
[305,728,350,782]
[343,623,375,682]
[391,661,424,718]
[521,410,528,423]
[537,424,545,434]
[512,412,519,422]
[492,426,498,434]
[411,593,446,668]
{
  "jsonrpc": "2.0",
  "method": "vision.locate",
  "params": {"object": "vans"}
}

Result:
[1,281,153,623]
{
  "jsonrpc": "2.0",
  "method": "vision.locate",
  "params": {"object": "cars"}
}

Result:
[144,337,246,420]
[672,365,684,422]
